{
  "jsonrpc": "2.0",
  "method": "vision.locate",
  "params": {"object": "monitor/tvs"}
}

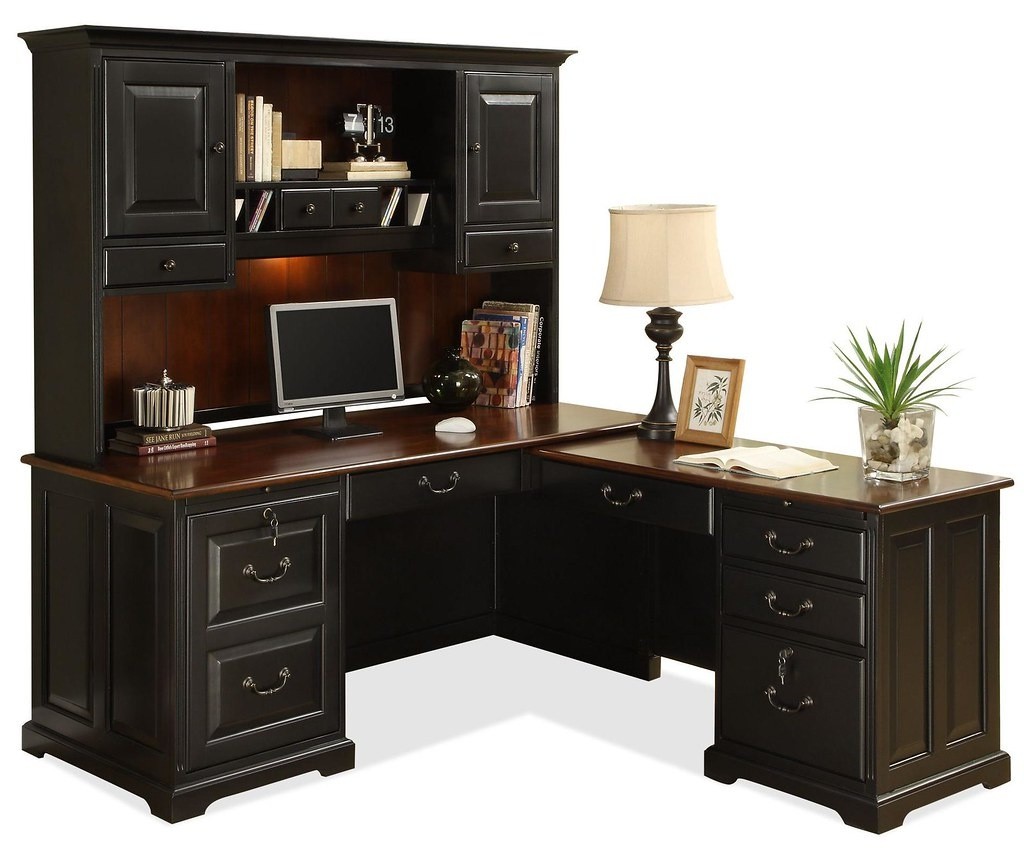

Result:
[264,297,405,440]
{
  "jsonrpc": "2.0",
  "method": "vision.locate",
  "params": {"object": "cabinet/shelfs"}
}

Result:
[17,26,1015,835]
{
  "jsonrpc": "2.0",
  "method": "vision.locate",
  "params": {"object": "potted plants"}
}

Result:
[831,323,970,479]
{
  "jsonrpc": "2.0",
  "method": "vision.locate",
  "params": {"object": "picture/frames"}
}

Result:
[674,355,745,449]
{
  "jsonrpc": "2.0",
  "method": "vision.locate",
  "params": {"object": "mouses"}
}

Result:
[434,416,476,432]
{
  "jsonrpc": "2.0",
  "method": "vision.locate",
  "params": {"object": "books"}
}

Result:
[106,422,217,457]
[380,187,403,227]
[671,445,839,481]
[317,161,412,180]
[407,192,429,226]
[461,299,548,409]
[234,92,282,183]
[235,190,274,233]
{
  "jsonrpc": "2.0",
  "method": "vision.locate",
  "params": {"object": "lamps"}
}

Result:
[600,206,733,442]
